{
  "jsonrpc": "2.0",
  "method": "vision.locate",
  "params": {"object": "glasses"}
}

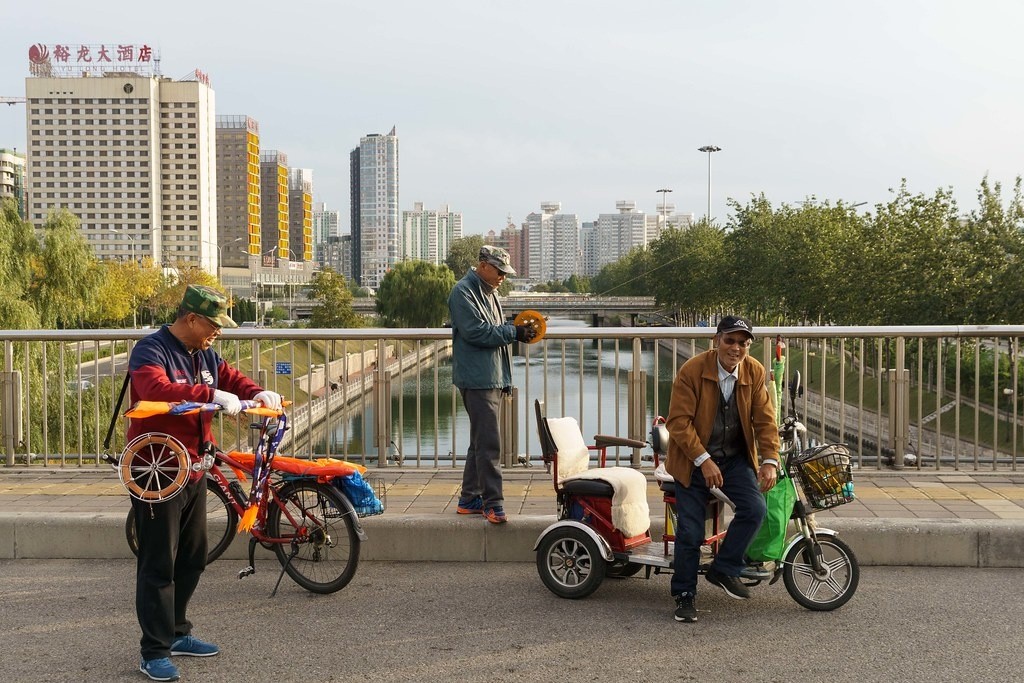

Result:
[722,337,749,348]
[202,319,221,333]
[494,266,504,276]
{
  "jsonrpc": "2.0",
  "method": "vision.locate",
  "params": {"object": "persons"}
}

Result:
[331,384,338,391]
[665,315,781,624]
[447,246,537,525]
[126,283,282,682]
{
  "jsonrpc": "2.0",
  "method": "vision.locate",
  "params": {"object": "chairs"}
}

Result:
[652,414,712,498]
[543,416,649,539]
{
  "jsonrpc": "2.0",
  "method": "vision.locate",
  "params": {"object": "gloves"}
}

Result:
[254,391,281,410]
[213,389,243,416]
[516,324,536,344]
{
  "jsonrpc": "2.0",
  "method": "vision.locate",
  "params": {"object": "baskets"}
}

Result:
[798,443,855,510]
[319,473,386,519]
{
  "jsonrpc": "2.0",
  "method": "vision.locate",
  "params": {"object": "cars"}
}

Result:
[66,381,95,394]
[241,316,311,328]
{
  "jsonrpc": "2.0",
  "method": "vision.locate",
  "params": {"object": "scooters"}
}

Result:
[533,369,860,613]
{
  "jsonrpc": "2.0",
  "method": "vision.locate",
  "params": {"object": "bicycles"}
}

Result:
[125,424,365,600]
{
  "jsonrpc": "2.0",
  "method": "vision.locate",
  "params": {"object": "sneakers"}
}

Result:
[170,635,218,657]
[673,596,698,621]
[483,505,508,523]
[706,566,752,600]
[456,496,483,513]
[140,657,181,681]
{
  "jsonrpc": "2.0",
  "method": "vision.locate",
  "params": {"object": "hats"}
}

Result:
[480,245,516,274]
[717,315,755,340]
[181,284,239,328]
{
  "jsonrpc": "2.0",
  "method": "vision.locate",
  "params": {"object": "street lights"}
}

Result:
[203,237,242,289]
[657,189,674,230]
[276,247,298,320]
[109,226,160,329]
[697,145,723,226]
[242,248,273,329]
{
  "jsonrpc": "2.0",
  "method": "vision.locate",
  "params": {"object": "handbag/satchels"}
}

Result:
[328,473,384,514]
